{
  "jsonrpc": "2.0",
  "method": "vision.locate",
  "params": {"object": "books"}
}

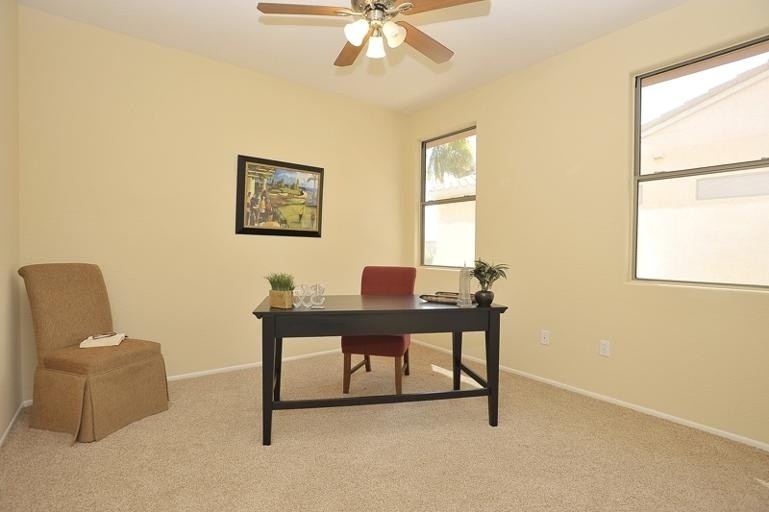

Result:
[78,331,127,347]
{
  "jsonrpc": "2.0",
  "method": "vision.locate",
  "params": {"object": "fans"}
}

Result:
[255,0,486,68]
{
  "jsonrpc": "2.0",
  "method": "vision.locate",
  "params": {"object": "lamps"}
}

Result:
[341,10,409,59]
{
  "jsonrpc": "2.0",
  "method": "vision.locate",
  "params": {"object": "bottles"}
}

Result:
[458,267,472,307]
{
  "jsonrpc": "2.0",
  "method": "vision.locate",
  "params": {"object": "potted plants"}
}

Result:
[262,273,298,310]
[468,257,511,305]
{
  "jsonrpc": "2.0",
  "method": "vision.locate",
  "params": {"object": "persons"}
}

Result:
[245,191,316,229]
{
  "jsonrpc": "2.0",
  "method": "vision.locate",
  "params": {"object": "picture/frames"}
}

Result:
[234,153,325,238]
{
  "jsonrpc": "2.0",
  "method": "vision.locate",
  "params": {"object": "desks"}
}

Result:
[249,293,509,448]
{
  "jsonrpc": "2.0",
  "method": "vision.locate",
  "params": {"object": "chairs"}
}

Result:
[17,262,172,445]
[340,265,417,398]
